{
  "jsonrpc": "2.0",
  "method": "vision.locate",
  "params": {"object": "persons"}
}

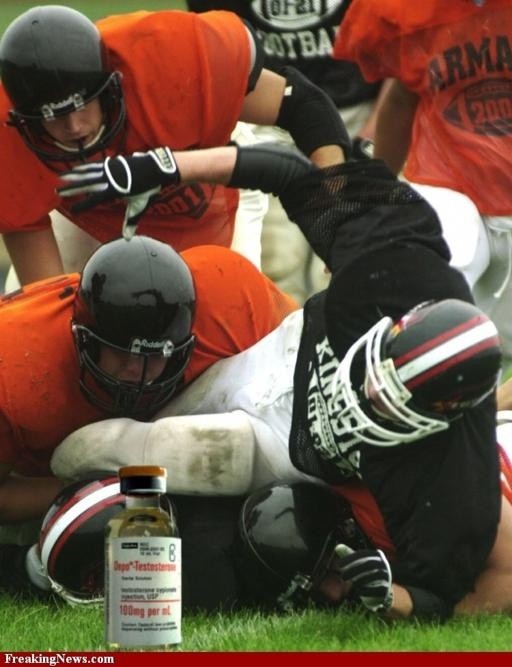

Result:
[2,1,512,626]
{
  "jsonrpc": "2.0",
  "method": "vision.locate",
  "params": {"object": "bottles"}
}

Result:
[103,466,184,644]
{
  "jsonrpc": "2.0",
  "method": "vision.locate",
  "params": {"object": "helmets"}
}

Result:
[74,237,197,425]
[39,472,171,604]
[1,6,129,176]
[329,298,501,449]
[239,478,351,609]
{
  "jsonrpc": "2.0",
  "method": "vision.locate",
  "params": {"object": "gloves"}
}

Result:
[335,543,394,615]
[57,146,181,240]
